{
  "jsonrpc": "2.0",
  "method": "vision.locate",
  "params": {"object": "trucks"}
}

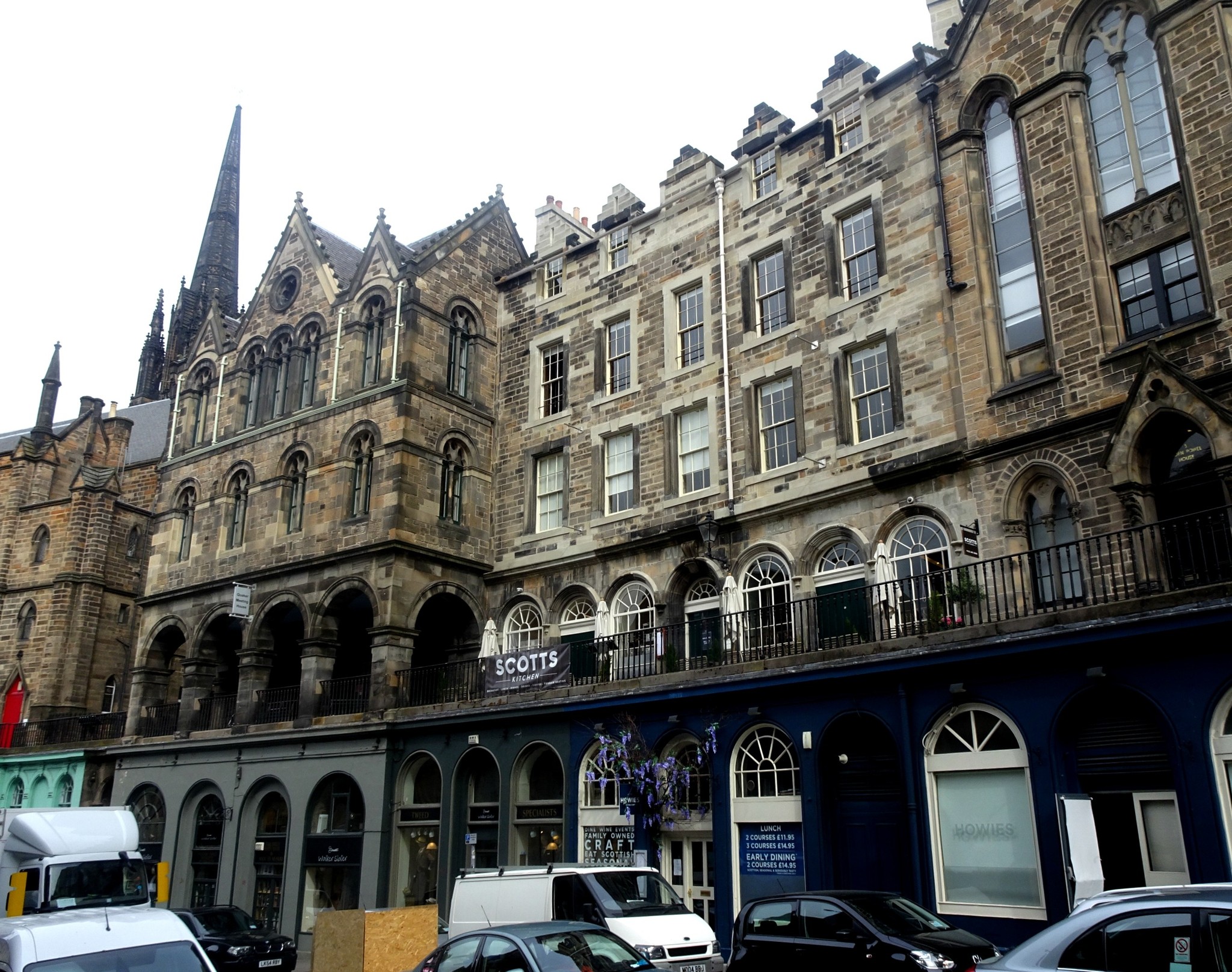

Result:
[0,798,169,917]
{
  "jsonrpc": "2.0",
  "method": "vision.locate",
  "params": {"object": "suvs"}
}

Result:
[166,904,298,971]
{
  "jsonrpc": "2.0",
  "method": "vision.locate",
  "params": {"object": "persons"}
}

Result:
[551,833,563,864]
[409,836,435,906]
[539,834,554,868]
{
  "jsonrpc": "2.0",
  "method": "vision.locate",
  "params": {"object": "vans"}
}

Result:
[450,862,724,971]
[1,908,216,972]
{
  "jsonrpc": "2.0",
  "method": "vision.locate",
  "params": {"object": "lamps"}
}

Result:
[697,509,729,565]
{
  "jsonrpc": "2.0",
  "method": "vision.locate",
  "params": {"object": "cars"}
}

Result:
[410,919,662,971]
[968,890,1232,972]
[727,881,998,972]
